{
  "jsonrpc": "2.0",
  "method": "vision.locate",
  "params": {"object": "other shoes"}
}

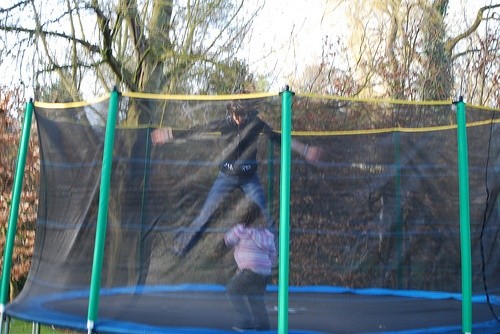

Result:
[173,239,186,257]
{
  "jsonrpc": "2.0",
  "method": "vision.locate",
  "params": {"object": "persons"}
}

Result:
[150,89,325,257]
[223,201,276,334]
